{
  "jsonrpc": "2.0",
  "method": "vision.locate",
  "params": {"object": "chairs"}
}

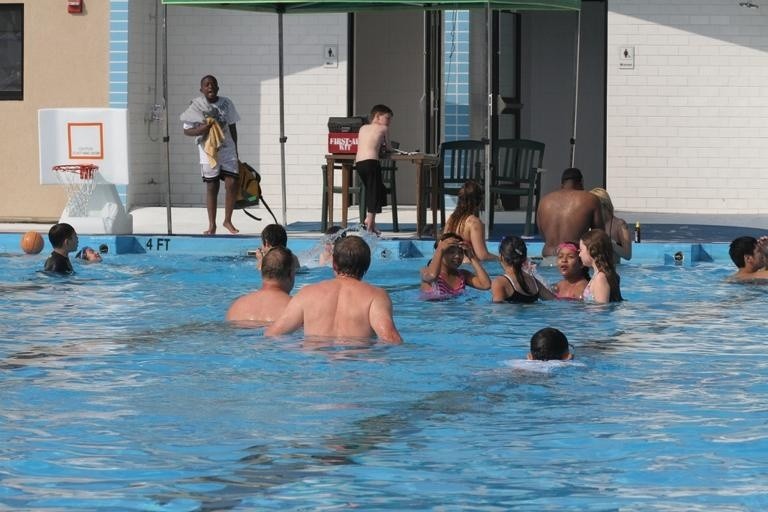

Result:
[422,139,490,231]
[320,138,402,235]
[479,136,546,239]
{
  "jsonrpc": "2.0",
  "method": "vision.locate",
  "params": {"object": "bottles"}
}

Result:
[633,218,641,244]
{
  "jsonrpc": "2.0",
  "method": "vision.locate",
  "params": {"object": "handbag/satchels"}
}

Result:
[233,160,260,208]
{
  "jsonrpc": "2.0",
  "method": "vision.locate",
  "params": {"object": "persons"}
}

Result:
[353,105,396,233]
[44,221,77,271]
[227,246,299,325]
[71,247,100,264]
[526,326,574,361]
[421,232,492,297]
[729,235,767,280]
[443,181,498,263]
[257,224,299,272]
[536,166,631,303]
[320,226,347,263]
[267,334,386,363]
[183,74,242,235]
[265,236,402,345]
[491,236,555,304]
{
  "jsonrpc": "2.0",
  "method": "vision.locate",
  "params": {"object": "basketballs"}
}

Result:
[21,231,43,254]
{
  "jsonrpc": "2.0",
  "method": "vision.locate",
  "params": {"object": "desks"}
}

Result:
[322,151,439,240]
